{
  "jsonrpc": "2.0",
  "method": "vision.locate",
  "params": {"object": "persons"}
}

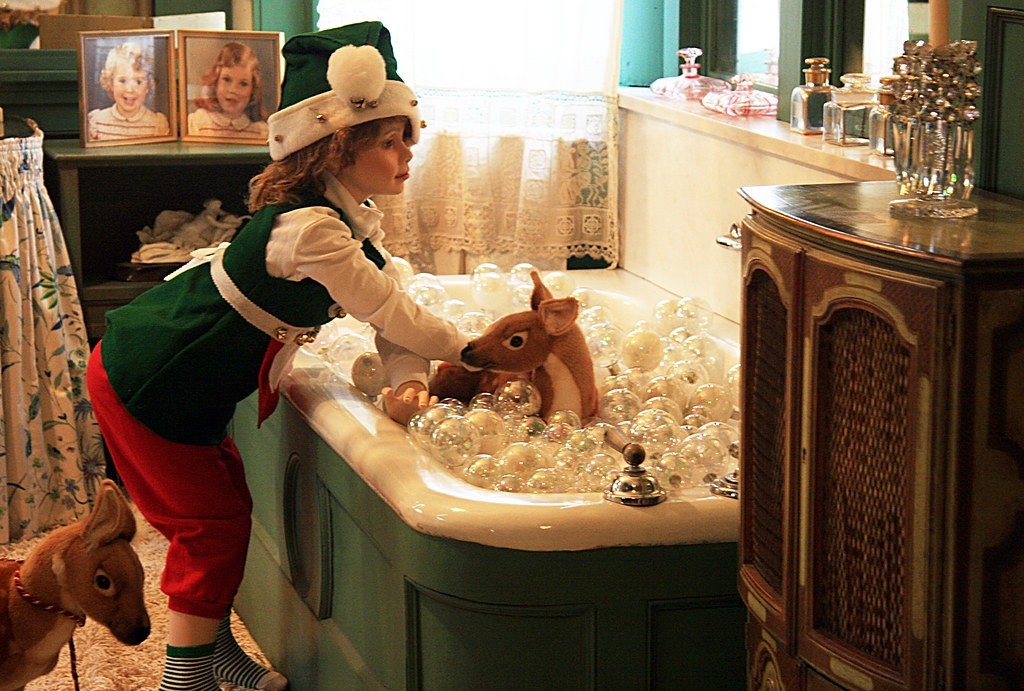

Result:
[187,43,270,136]
[87,21,468,691]
[88,43,171,142]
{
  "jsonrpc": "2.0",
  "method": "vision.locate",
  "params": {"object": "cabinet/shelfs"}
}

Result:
[735,177,1024,691]
[42,135,278,353]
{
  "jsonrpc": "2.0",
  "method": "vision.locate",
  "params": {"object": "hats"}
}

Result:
[267,21,429,161]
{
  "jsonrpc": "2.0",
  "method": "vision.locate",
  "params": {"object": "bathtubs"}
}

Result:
[234,268,743,691]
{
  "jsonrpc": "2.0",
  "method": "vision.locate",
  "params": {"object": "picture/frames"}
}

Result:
[177,28,283,148]
[76,28,177,149]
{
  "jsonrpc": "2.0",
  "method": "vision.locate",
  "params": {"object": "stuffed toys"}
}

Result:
[0,478,150,691]
[429,270,598,423]
